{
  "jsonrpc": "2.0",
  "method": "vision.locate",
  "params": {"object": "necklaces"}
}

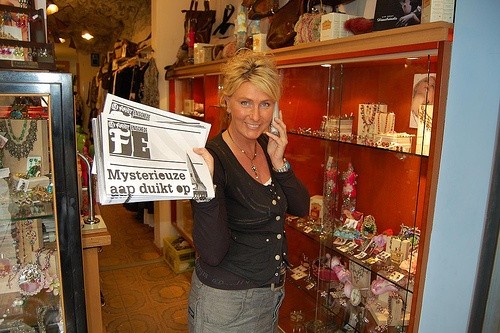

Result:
[390,237,403,250]
[0,119,38,162]
[0,220,55,270]
[361,104,377,125]
[378,113,388,134]
[227,130,258,178]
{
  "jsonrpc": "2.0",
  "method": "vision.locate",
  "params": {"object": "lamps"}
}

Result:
[46,0,58,15]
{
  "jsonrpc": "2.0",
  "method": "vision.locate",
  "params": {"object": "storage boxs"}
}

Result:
[164,237,195,273]
[421,0,455,23]
[320,13,360,41]
[253,34,266,52]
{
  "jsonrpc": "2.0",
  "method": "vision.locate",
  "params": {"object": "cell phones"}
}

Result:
[270,101,279,136]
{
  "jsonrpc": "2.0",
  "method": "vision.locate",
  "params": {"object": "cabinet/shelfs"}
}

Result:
[0,0,88,333]
[165,21,454,332]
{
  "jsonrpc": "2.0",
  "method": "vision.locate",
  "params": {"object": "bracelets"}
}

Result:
[273,159,290,172]
[361,221,377,239]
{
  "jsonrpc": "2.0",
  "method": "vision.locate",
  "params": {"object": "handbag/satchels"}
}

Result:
[247,0,279,20]
[181,0,216,50]
[293,0,327,45]
[266,0,313,49]
[101,72,111,89]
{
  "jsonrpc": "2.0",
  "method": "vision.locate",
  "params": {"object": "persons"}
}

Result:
[395,0,420,26]
[187,51,311,333]
[410,76,436,127]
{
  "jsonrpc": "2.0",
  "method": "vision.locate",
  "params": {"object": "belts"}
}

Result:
[258,272,286,292]
